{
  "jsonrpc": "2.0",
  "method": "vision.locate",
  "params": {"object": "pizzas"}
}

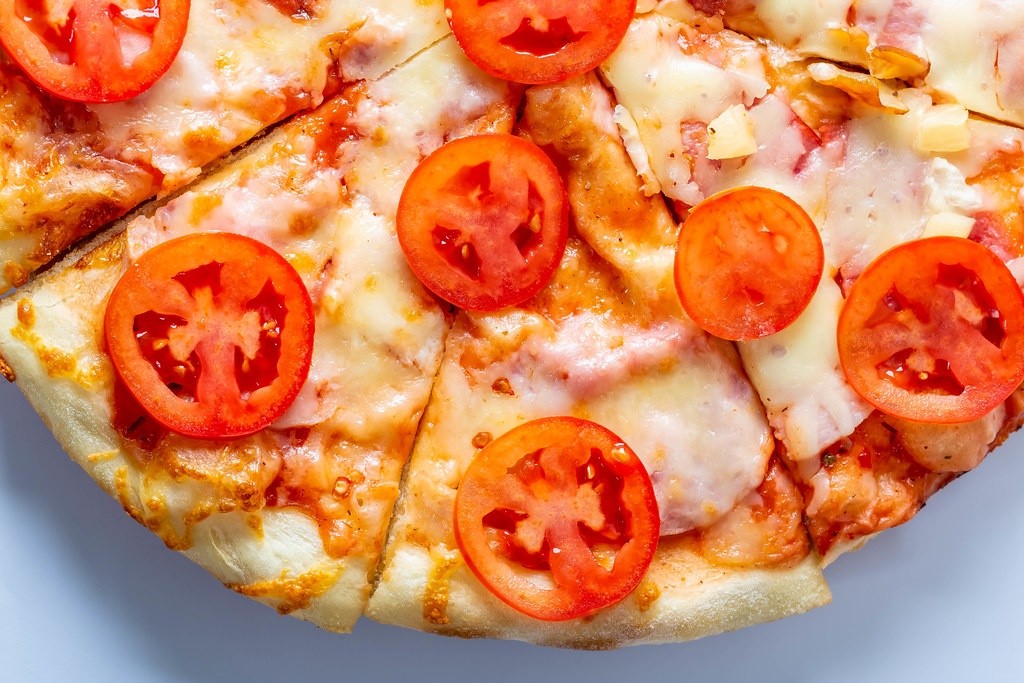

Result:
[0,0,1024,652]
